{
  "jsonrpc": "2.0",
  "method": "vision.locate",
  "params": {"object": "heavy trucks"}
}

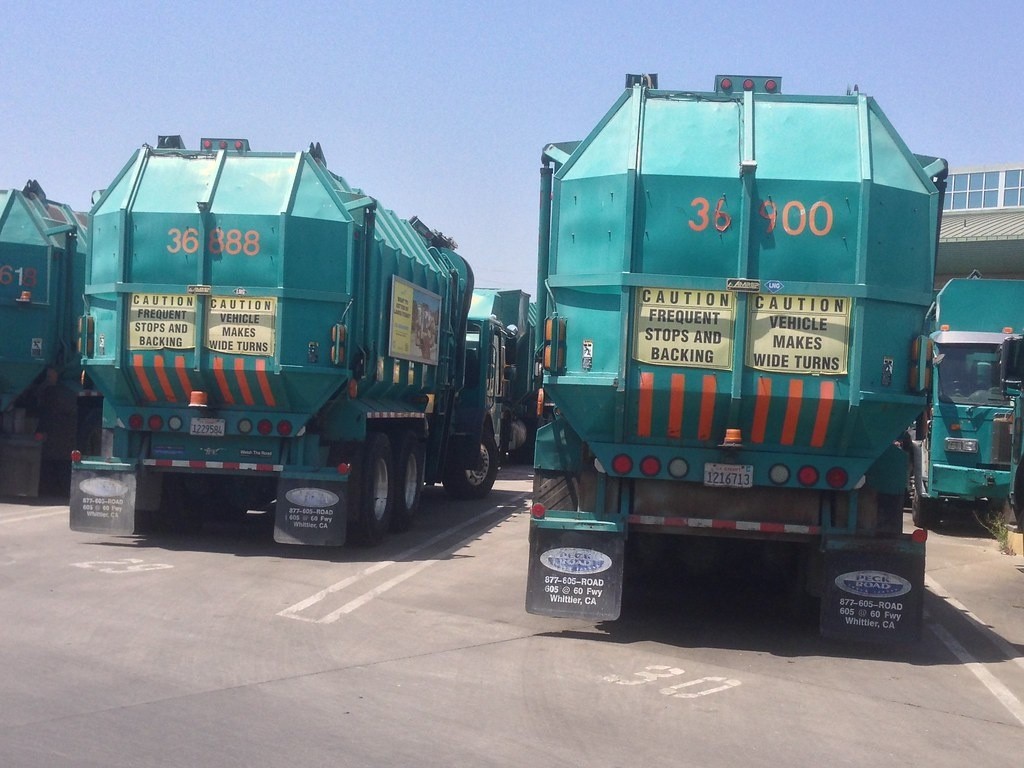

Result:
[909,271,1022,537]
[537,72,954,653]
[72,135,498,551]
[471,287,540,465]
[1,179,103,500]
